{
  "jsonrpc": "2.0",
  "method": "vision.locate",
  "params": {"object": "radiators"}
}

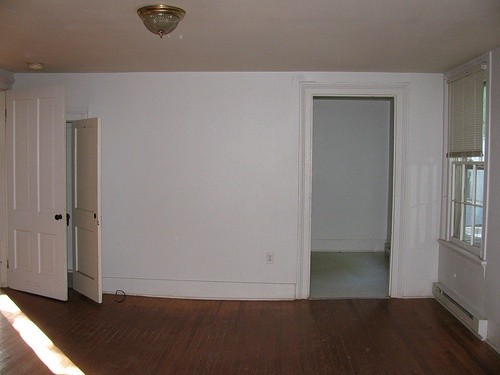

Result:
[430,283,489,340]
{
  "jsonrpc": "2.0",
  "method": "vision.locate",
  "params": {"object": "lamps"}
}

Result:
[137,4,185,37]
